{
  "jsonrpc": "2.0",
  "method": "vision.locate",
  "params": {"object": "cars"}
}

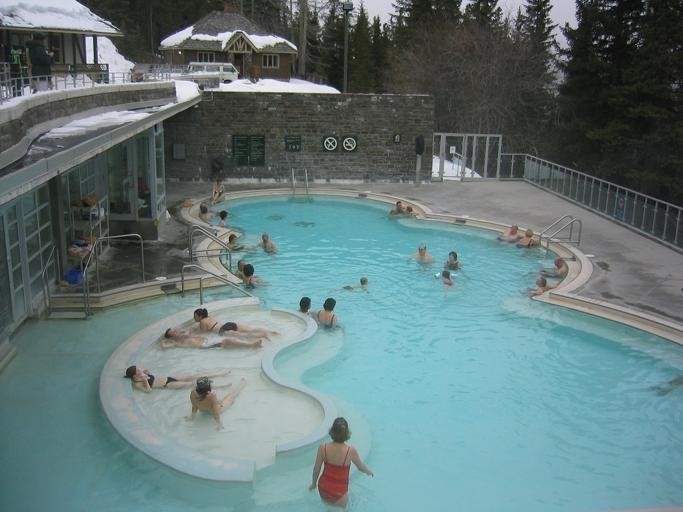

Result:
[130,64,156,83]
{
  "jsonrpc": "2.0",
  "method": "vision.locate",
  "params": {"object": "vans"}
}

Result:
[181,62,241,83]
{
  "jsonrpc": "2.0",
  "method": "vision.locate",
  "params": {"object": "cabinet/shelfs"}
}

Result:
[66,194,110,274]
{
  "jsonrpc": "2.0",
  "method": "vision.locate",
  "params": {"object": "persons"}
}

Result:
[4,35,23,95]
[296,296,311,317]
[315,297,338,328]
[542,257,568,279]
[199,178,279,289]
[26,33,54,95]
[442,270,455,297]
[389,201,406,215]
[344,276,369,292]
[406,206,419,216]
[519,276,557,296]
[443,251,463,274]
[412,242,434,264]
[516,229,539,257]
[309,416,374,507]
[496,223,525,244]
[123,308,280,431]
